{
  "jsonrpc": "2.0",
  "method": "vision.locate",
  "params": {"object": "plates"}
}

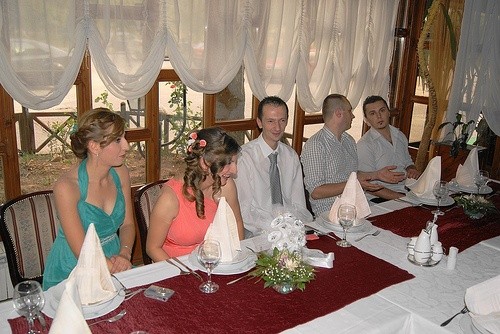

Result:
[407,191,455,206]
[315,210,372,233]
[450,186,493,194]
[471,313,500,334]
[41,274,125,320]
[198,246,258,275]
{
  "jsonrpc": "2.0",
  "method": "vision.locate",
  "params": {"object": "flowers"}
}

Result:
[190,132,197,139]
[188,147,191,151]
[454,192,495,211]
[251,212,317,287]
[200,140,206,146]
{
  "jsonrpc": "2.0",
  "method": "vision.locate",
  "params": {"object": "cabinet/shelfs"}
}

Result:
[434,143,487,182]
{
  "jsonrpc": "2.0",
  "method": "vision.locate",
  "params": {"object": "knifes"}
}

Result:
[171,255,203,281]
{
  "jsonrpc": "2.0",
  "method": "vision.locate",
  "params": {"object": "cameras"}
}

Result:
[143,284,175,302]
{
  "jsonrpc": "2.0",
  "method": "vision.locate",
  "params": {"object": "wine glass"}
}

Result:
[474,170,489,196]
[12,280,45,334]
[431,180,448,216]
[197,240,220,293]
[335,203,357,247]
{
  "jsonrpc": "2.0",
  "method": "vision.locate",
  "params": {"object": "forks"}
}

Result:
[355,229,382,242]
[88,309,128,326]
[440,307,468,327]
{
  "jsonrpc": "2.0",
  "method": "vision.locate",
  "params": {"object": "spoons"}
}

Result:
[166,259,191,275]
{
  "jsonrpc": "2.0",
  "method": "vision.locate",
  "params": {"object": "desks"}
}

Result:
[0,176,500,334]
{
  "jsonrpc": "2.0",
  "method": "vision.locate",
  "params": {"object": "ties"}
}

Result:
[267,153,284,207]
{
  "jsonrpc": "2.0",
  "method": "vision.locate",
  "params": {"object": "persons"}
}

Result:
[146,127,244,262]
[232,96,313,238]
[301,94,406,220]
[43,108,136,290]
[357,96,419,203]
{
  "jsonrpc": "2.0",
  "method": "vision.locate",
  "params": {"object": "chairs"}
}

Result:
[0,190,59,295]
[136,180,170,264]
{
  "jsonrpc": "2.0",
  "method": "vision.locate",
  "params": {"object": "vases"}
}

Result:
[463,208,488,219]
[274,283,295,294]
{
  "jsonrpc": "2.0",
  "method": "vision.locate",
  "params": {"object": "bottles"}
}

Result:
[409,223,445,263]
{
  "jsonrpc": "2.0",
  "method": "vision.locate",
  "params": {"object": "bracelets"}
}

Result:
[121,246,132,253]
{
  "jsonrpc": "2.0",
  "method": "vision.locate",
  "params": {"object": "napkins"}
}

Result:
[66,222,116,305]
[204,196,241,262]
[404,156,441,200]
[47,288,91,334]
[330,172,371,224]
[456,147,481,185]
